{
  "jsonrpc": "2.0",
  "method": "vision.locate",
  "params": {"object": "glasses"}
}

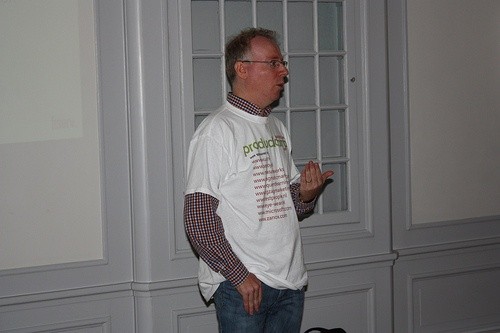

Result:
[236,58,288,70]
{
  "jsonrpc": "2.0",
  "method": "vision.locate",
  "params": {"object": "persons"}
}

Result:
[180,26,335,333]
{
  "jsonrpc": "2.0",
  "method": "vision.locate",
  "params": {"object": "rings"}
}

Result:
[306,179,312,183]
